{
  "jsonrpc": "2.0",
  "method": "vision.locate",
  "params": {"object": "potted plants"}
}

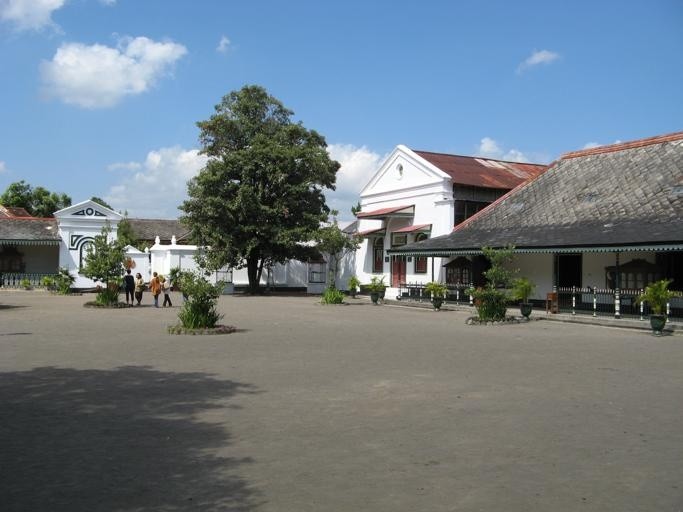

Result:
[506,276,539,317]
[422,282,448,311]
[168,268,182,291]
[19,279,30,290]
[632,278,682,338]
[345,275,387,305]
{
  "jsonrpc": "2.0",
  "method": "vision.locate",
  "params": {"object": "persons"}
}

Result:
[161,278,172,306]
[124,269,134,307]
[134,273,145,305]
[147,271,161,307]
